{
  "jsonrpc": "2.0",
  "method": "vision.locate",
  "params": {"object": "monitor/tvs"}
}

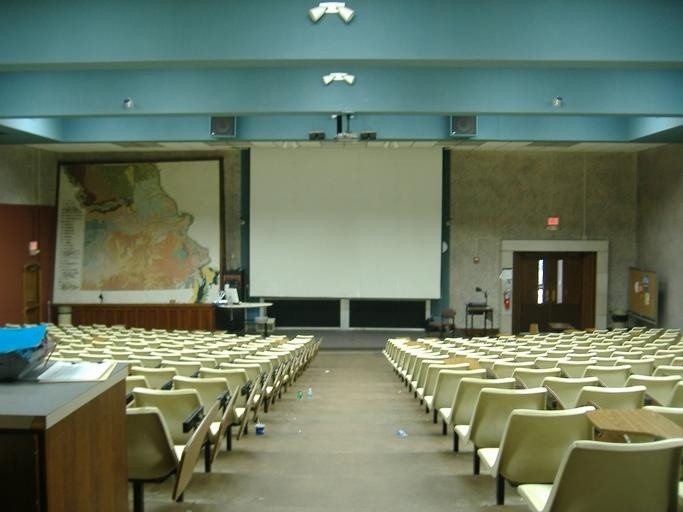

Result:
[221,271,244,302]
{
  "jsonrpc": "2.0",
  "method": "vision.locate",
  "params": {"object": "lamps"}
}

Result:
[309,2,354,24]
[323,70,355,86]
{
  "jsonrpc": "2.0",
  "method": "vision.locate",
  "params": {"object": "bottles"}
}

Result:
[306,387,313,400]
[396,429,408,440]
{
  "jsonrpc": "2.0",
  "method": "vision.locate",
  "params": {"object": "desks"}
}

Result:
[216,303,274,336]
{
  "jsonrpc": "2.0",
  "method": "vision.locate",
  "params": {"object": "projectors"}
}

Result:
[334,134,356,143]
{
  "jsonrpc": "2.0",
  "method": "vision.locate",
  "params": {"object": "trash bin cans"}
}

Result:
[57,306,71,325]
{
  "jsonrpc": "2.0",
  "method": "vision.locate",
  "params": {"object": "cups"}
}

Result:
[254,424,264,435]
[296,390,303,400]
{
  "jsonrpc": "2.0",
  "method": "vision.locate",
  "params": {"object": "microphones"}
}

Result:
[474,286,481,293]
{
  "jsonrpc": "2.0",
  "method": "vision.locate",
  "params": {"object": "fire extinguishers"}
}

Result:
[504,289,510,309]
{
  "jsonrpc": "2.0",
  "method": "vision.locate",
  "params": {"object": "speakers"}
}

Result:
[308,131,326,141]
[362,133,378,140]
[449,115,478,138]
[210,116,236,137]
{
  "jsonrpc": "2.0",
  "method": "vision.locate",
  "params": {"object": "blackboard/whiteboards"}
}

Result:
[627,267,659,324]
